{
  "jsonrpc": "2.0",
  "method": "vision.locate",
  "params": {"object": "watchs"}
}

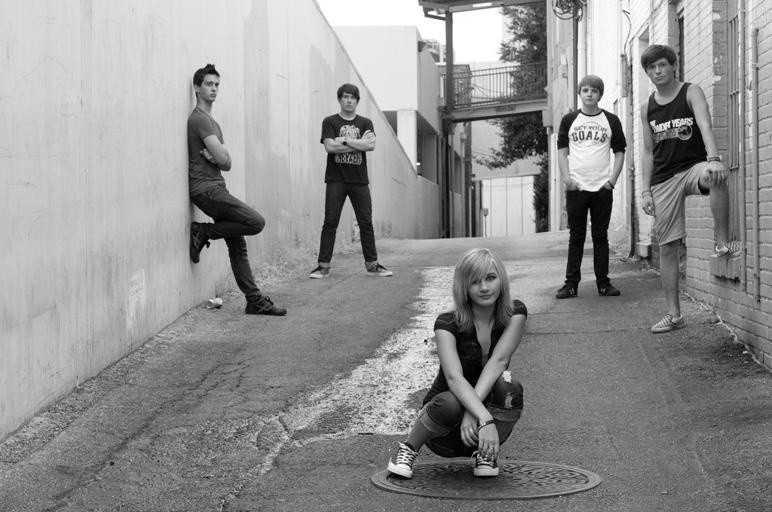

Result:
[343,137,347,146]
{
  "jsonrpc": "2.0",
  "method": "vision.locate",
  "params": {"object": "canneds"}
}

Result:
[206,298,223,309]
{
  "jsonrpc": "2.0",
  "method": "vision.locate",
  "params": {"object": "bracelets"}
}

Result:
[477,420,495,432]
[608,180,615,189]
[706,157,720,163]
[642,191,652,198]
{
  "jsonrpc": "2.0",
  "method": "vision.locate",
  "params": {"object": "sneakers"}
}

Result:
[364,261,393,277]
[597,281,622,296]
[387,444,418,479]
[309,266,330,279]
[555,283,578,299]
[651,314,685,332]
[473,449,501,477]
[190,221,206,263]
[713,241,743,256]
[244,296,287,316]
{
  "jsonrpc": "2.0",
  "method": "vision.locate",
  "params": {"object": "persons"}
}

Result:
[309,83,393,280]
[386,247,527,480]
[187,64,287,316]
[555,76,627,299]
[641,44,741,333]
